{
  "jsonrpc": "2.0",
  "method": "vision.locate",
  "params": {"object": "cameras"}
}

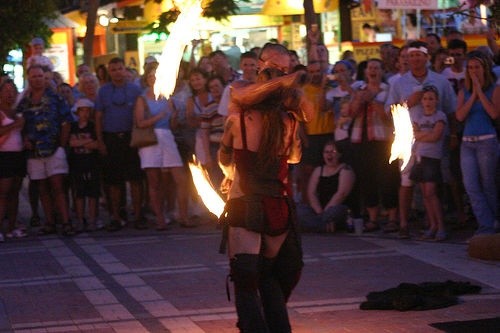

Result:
[443,57,455,64]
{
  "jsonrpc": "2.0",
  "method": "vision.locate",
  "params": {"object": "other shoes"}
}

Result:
[30,216,42,226]
[0,228,30,242]
[37,209,198,236]
[362,221,449,243]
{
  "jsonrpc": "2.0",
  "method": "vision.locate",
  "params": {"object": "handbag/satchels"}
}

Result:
[129,96,158,149]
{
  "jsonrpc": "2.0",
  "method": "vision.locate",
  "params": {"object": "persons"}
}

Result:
[461,11,489,33]
[363,23,377,42]
[0,72,28,241]
[303,29,500,243]
[401,14,426,40]
[14,57,142,236]
[26,37,58,91]
[217,42,306,332]
[303,22,322,45]
[174,38,306,227]
[134,56,199,231]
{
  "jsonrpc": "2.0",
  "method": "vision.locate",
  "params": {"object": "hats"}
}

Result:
[144,56,159,65]
[28,38,43,46]
[70,99,95,114]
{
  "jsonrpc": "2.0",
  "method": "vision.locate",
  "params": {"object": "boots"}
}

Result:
[227,254,292,333]
